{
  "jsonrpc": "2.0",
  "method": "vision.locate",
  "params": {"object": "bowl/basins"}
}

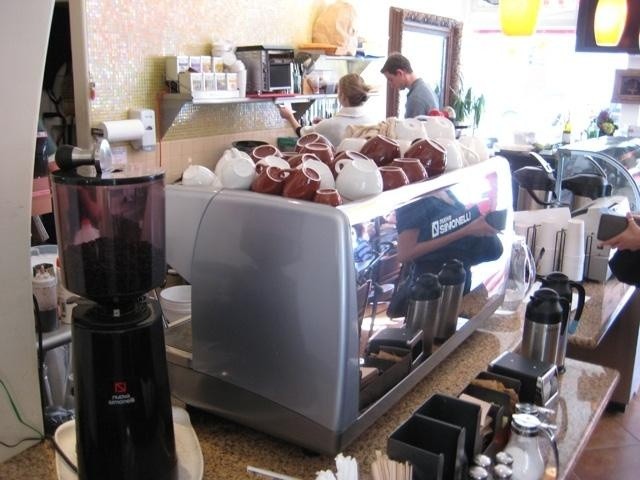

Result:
[159,284,191,323]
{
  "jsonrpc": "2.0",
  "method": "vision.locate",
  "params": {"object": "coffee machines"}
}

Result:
[49,138,178,480]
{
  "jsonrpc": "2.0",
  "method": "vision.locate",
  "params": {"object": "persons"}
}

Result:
[391,187,508,314]
[351,210,401,283]
[596,207,638,256]
[380,52,435,119]
[279,72,382,150]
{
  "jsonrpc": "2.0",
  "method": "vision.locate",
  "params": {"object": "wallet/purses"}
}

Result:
[597,215,639,240]
[485,209,507,229]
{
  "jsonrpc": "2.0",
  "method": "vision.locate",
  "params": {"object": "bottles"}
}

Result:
[520,272,586,378]
[32,257,80,334]
[502,413,559,480]
[561,111,573,145]
[405,258,467,360]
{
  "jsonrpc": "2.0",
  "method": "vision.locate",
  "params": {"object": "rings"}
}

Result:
[627,218,634,222]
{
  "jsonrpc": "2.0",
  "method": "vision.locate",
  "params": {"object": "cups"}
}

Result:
[536,218,587,282]
[180,116,490,207]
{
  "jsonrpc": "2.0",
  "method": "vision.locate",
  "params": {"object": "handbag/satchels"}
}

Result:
[386,261,416,319]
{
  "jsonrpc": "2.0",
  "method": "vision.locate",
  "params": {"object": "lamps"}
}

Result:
[499,0,541,37]
[593,0,628,48]
